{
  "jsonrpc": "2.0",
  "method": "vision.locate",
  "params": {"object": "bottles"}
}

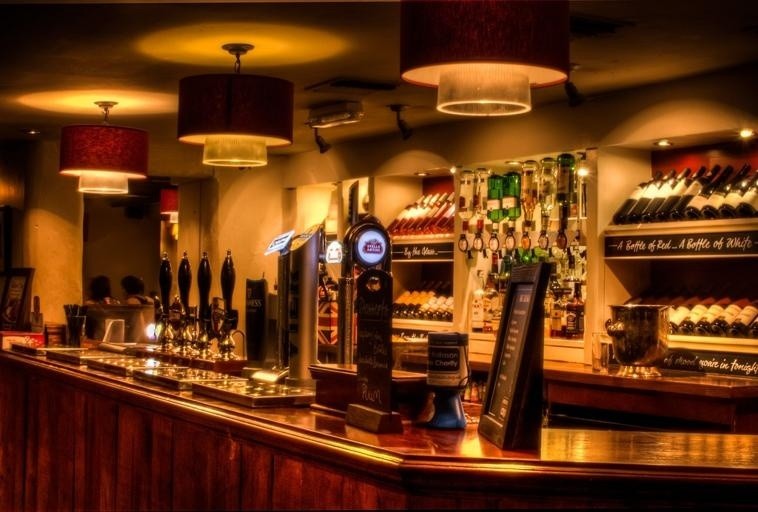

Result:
[611,163,758,224]
[624,287,757,339]
[470,241,584,338]
[457,153,576,240]
[386,192,455,235]
[394,277,454,322]
[460,382,487,403]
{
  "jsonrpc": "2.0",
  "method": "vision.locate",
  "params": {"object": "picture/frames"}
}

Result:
[476,262,552,451]
[0,267,36,331]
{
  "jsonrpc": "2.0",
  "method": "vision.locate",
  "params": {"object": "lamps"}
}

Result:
[399,0,569,117]
[564,80,585,106]
[304,121,333,154]
[386,104,415,139]
[177,43,295,168]
[58,101,148,195]
[159,188,179,224]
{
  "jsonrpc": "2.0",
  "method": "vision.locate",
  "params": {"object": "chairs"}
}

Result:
[90,276,118,301]
[121,276,145,297]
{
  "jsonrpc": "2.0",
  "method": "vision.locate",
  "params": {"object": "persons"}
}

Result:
[80,275,162,343]
